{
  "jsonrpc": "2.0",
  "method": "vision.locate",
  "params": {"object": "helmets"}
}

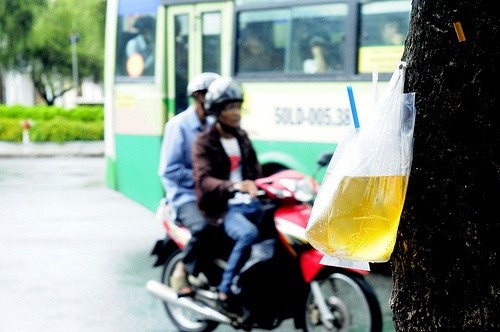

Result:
[204,77,244,111]
[187,72,219,97]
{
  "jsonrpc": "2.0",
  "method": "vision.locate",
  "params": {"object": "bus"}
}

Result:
[102,0,413,276]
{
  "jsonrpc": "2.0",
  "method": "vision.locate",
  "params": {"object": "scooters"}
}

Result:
[142,153,384,332]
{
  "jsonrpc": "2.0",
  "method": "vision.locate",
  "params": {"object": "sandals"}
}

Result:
[169,276,194,297]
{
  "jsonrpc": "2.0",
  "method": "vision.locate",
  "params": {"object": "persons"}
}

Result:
[380,15,405,45]
[303,37,341,73]
[125,14,189,93]
[159,72,235,296]
[192,76,276,318]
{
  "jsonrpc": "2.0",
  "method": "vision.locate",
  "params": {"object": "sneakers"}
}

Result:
[216,298,244,317]
